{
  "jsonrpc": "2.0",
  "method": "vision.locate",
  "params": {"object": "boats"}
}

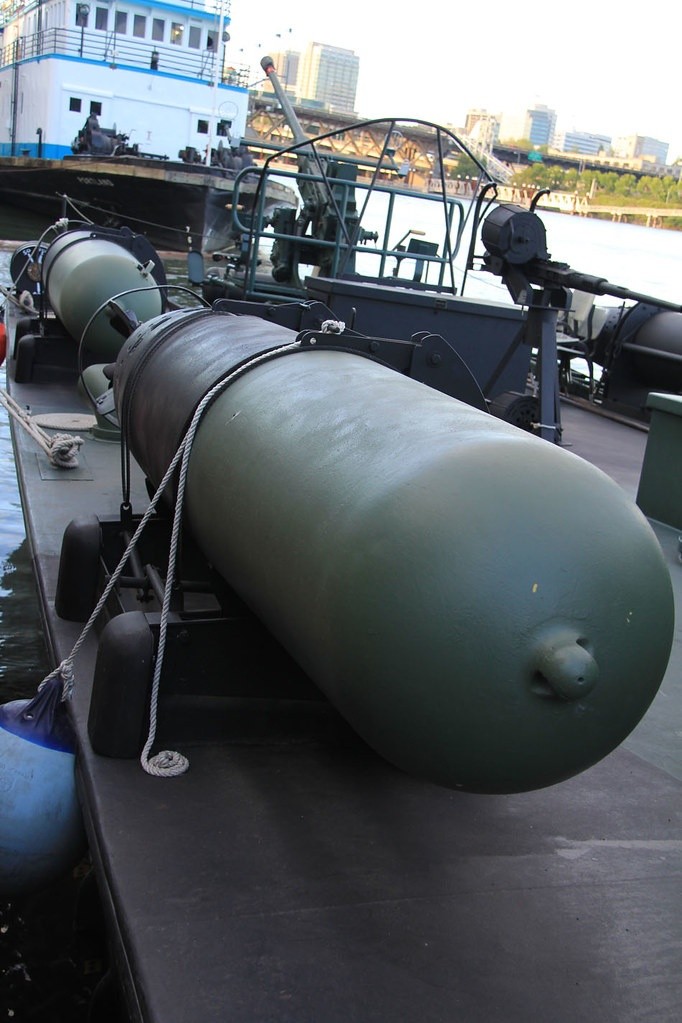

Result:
[0,0,298,253]
[0,56,682,1023]
